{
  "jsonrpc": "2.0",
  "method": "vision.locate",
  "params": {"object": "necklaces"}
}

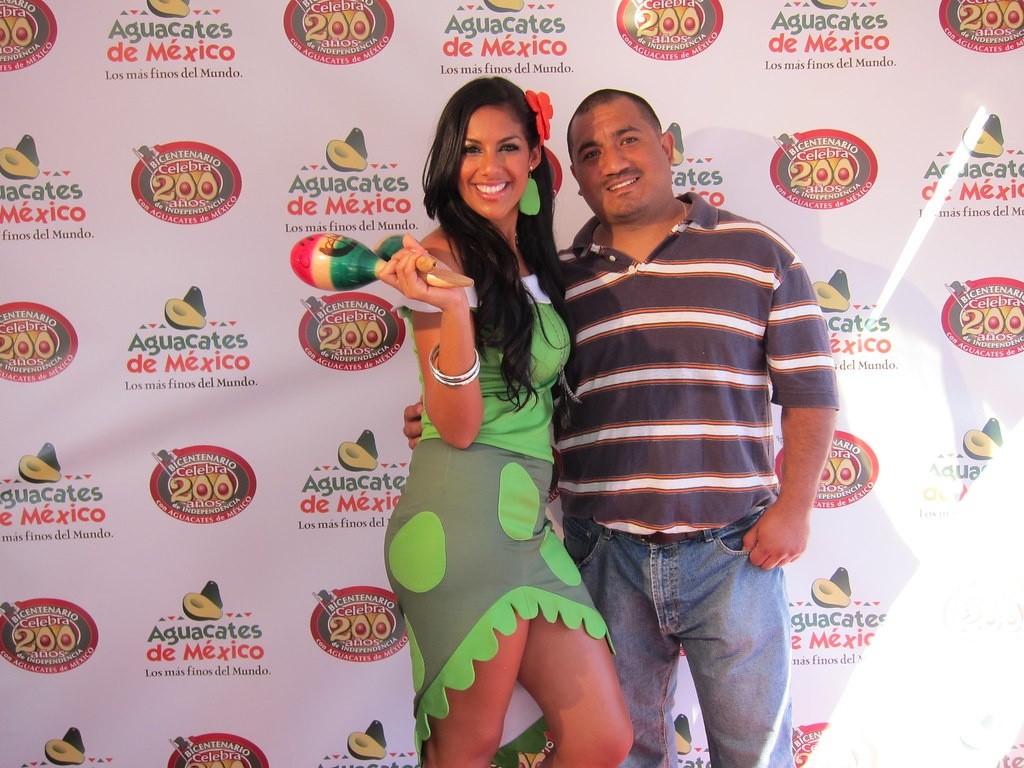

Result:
[678,199,688,218]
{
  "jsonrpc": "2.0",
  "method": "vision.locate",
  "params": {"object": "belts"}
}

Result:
[590,516,720,545]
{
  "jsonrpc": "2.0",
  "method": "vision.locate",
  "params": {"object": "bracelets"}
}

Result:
[428,342,481,386]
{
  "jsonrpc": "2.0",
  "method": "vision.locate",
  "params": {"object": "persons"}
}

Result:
[403,89,840,768]
[374,76,633,768]
